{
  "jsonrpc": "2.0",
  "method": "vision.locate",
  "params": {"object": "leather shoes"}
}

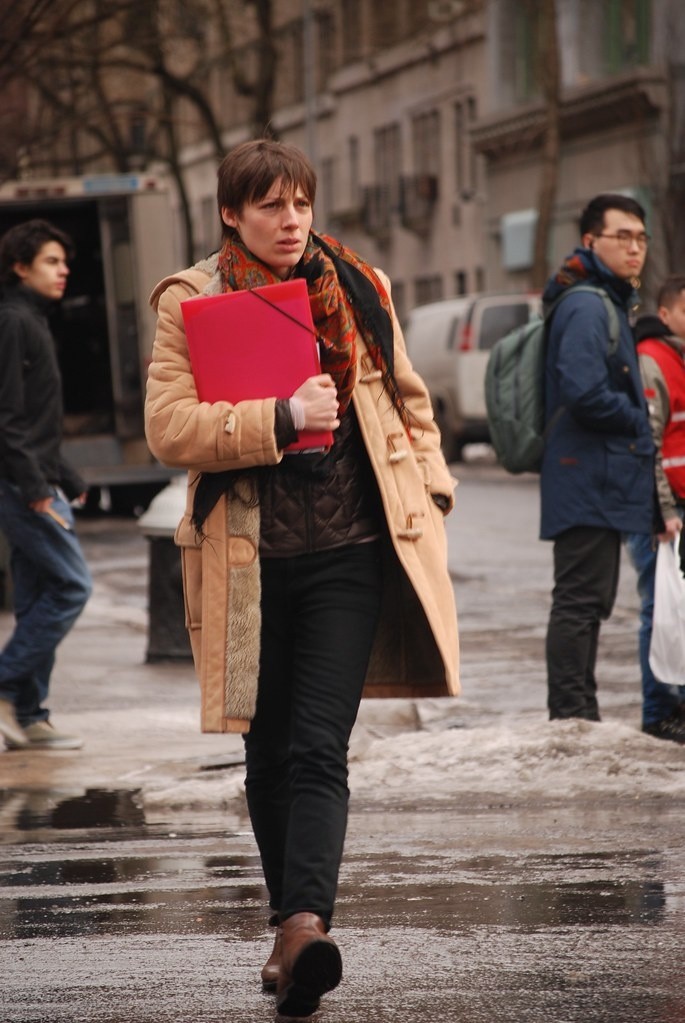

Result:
[277,913,342,1020]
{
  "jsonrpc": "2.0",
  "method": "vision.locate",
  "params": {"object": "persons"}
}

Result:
[537,195,655,722]
[0,221,95,748]
[141,140,459,1017]
[627,275,685,744]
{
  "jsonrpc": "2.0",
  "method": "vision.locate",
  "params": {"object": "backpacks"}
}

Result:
[482,286,620,475]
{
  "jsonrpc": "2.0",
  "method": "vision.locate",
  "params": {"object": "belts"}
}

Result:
[261,924,281,989]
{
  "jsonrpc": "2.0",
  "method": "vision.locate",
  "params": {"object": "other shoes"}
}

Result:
[642,714,685,744]
[0,700,29,747]
[5,720,83,749]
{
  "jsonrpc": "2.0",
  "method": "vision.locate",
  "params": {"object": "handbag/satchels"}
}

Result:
[649,531,685,685]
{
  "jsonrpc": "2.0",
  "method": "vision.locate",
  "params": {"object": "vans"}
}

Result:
[395,294,542,464]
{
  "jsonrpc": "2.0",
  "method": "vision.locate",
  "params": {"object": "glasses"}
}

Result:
[591,230,652,252]
